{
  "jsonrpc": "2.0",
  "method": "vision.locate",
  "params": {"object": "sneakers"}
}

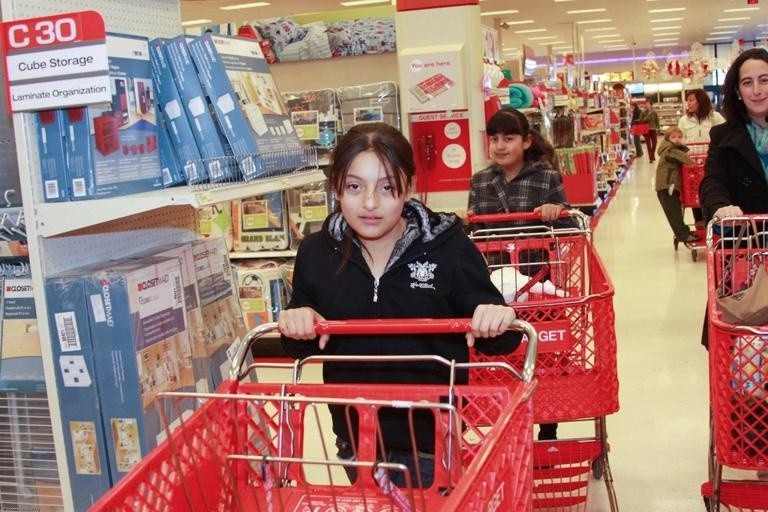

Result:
[682,233,702,242]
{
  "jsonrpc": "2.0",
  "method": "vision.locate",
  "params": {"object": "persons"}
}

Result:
[638,99,660,164]
[627,103,643,158]
[653,125,702,243]
[676,87,726,230]
[273,121,517,490]
[700,46,767,482]
[452,104,581,470]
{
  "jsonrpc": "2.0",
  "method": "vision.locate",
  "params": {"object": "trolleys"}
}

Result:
[86,316,537,512]
[672,141,708,262]
[701,212,768,512]
[465,210,620,512]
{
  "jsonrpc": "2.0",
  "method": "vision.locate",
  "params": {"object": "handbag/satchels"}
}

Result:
[715,216,768,327]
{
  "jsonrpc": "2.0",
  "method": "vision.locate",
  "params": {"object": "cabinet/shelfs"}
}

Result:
[493,73,681,224]
[0,0,394,512]
